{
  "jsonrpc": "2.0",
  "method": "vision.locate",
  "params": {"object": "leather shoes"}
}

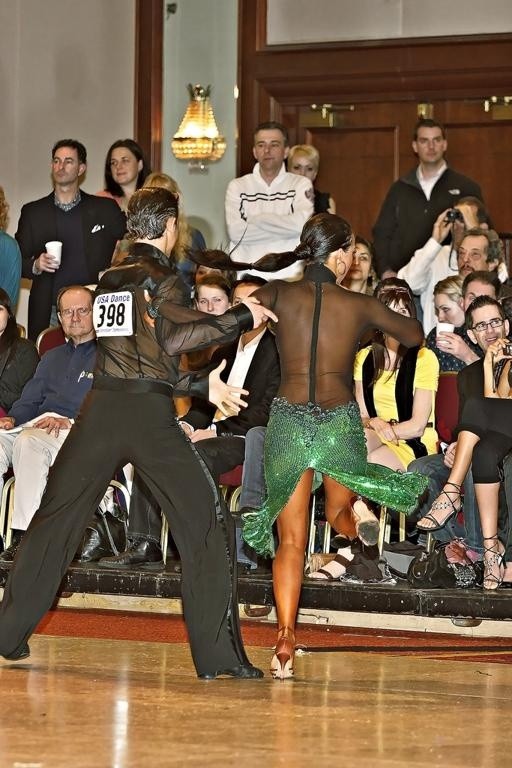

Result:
[100,539,165,571]
[201,663,266,681]
[0,532,23,564]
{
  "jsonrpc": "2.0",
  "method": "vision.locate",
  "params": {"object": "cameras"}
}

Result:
[502,343,511,357]
[444,207,463,225]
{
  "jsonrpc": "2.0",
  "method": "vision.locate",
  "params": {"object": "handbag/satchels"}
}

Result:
[73,504,126,564]
[406,541,485,590]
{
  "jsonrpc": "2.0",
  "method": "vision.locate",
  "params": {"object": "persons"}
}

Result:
[143,209,423,681]
[0,185,285,679]
[3,121,512,595]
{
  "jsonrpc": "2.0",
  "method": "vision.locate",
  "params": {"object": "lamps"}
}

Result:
[171,83,227,173]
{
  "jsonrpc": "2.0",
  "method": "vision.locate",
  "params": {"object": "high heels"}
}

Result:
[483,534,507,591]
[349,495,380,546]
[270,626,296,683]
[416,481,463,531]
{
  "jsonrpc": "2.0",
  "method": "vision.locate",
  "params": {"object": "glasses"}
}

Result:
[59,307,92,317]
[380,287,413,301]
[472,318,504,332]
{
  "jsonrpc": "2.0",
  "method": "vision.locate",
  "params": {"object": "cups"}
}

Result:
[44,241,62,268]
[434,323,454,350]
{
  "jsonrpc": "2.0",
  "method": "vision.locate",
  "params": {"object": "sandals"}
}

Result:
[307,554,350,582]
[328,534,362,549]
